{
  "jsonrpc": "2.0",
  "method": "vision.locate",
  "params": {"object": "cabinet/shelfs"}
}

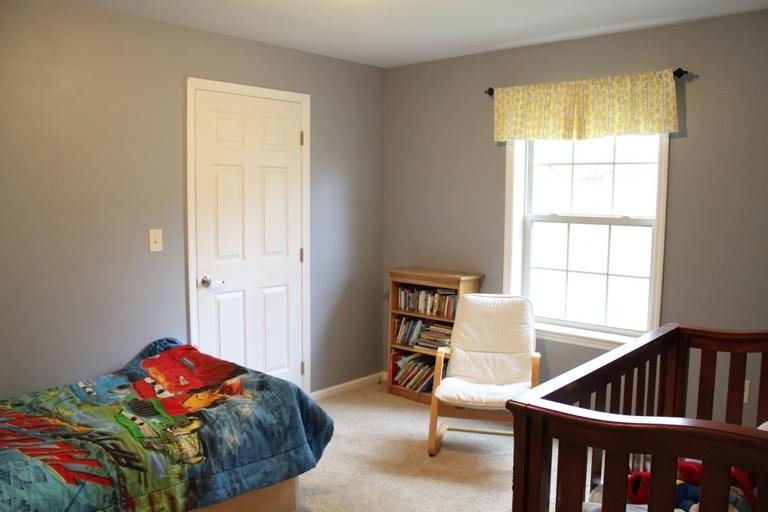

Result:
[379,267,484,407]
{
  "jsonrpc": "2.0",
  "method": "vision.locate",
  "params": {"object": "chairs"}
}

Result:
[427,293,541,457]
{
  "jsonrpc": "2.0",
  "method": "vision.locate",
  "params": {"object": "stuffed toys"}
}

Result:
[628,471,753,512]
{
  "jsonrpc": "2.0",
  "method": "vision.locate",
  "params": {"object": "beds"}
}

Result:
[505,322,768,512]
[0,336,333,511]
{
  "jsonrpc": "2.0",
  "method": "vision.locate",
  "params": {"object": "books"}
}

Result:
[395,317,453,349]
[398,287,456,320]
[394,353,447,393]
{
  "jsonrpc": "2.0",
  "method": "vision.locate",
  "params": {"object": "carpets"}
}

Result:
[298,383,604,512]
